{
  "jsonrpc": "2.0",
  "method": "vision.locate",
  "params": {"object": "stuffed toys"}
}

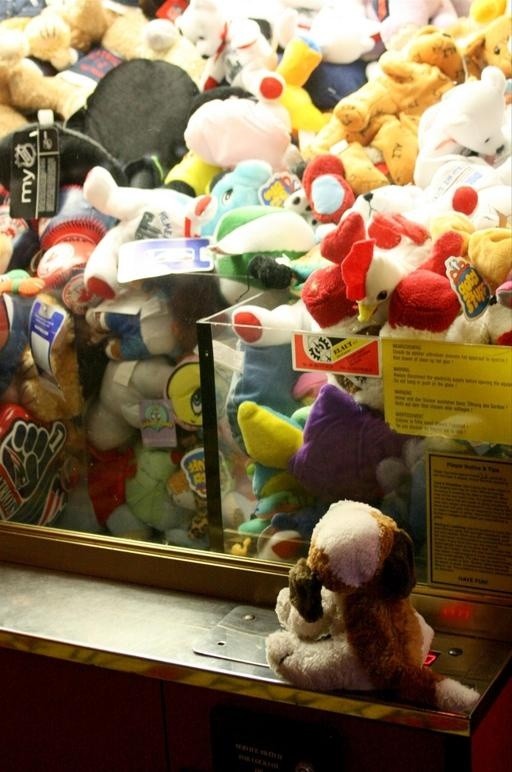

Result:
[1,1,512,571]
[1,2,512,573]
[263,495,476,708]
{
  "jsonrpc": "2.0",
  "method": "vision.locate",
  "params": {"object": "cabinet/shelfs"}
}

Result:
[0,290,511,770]
